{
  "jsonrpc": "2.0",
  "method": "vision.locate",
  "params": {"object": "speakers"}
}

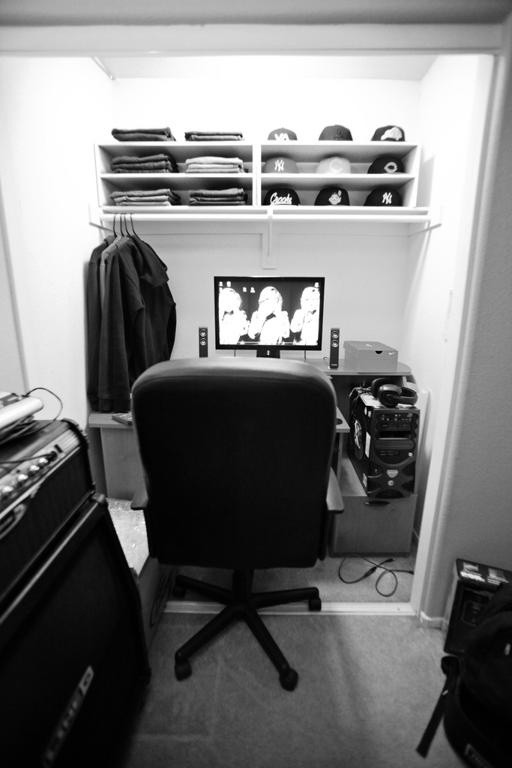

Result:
[198,327,208,355]
[330,327,339,367]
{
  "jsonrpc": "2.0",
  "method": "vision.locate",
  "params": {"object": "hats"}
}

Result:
[262,125,405,207]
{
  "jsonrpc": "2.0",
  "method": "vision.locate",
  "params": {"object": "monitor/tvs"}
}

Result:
[214,274,327,364]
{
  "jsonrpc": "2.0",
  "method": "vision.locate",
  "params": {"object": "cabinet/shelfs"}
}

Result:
[91,391,350,508]
[96,134,429,231]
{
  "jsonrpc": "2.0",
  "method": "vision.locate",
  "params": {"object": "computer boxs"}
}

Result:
[348,381,421,497]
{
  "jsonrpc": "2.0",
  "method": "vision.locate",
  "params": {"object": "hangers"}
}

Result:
[94,213,147,258]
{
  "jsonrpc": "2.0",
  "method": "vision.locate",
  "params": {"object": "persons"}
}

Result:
[247,285,291,344]
[290,284,320,346]
[219,287,250,344]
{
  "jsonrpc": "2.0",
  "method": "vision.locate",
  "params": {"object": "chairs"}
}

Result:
[130,358,346,691]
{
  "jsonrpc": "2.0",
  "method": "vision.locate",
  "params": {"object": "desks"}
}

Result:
[283,340,412,378]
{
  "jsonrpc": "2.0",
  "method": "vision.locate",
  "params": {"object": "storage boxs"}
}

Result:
[103,498,175,647]
[330,447,418,557]
[343,338,399,372]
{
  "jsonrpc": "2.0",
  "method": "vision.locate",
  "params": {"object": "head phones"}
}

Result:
[371,377,419,408]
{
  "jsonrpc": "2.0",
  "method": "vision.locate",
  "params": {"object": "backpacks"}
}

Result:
[442,581,512,768]
